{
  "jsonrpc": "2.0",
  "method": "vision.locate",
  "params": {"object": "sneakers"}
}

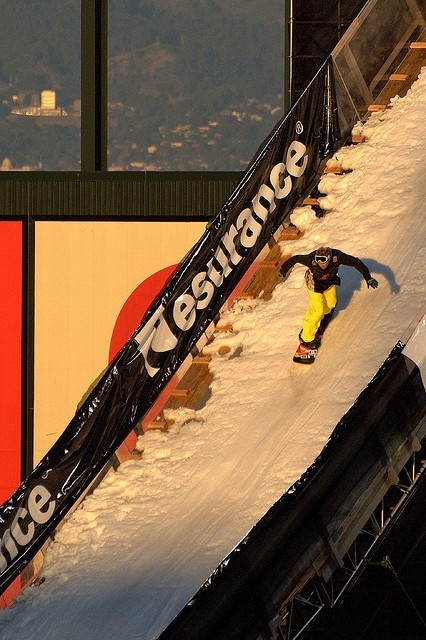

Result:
[299,329,320,350]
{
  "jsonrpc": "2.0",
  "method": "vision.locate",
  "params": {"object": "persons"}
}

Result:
[278,247,379,349]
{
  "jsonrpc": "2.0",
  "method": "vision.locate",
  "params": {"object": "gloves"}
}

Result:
[279,268,288,278]
[367,278,379,289]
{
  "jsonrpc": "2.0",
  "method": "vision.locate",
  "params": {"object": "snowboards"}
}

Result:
[292,301,338,365]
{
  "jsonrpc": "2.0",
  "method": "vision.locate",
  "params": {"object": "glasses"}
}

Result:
[315,255,331,264]
